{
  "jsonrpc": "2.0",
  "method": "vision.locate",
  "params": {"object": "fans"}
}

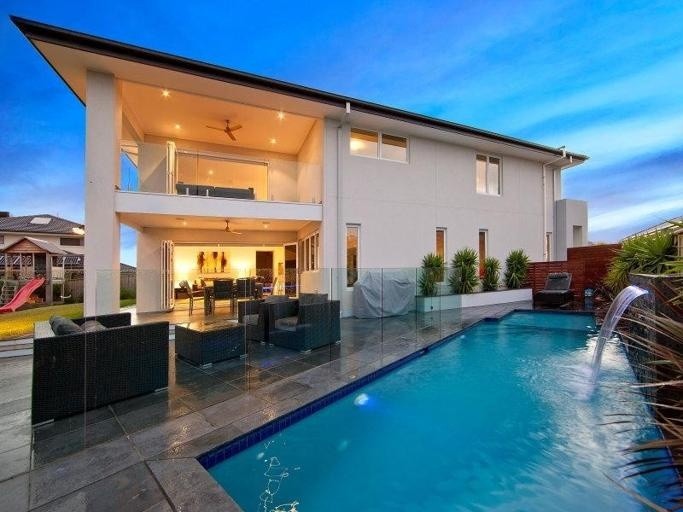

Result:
[206,118,242,141]
[205,218,247,240]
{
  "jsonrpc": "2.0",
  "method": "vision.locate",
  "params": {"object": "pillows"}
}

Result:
[262,292,329,305]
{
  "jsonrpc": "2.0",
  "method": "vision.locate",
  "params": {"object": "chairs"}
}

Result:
[237,298,340,354]
[532,271,576,308]
[178,277,256,317]
[31,310,173,426]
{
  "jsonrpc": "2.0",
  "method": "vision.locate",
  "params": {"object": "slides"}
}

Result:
[0,278,45,314]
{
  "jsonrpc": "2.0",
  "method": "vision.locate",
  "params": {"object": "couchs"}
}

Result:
[176,183,254,200]
